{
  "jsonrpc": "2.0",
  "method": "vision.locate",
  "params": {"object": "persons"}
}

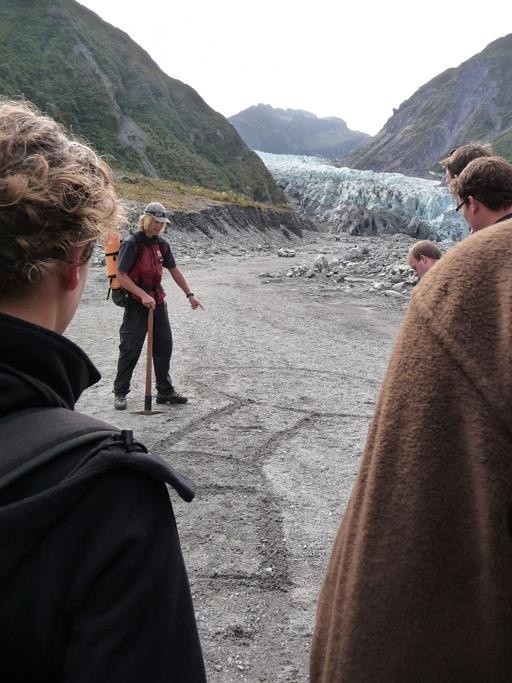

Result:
[113,202,207,408]
[2,94,209,680]
[452,155,512,235]
[408,240,443,281]
[437,141,493,203]
[311,220,512,683]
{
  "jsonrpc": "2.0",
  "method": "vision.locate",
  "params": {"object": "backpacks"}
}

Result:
[106,229,165,308]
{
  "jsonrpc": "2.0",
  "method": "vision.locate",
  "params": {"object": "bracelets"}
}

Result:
[186,294,195,299]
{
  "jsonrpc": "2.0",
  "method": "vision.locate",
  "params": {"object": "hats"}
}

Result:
[144,201,171,223]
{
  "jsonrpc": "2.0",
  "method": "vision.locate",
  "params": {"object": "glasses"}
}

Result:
[456,194,474,215]
[144,210,164,218]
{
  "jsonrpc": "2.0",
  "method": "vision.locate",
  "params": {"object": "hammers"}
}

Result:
[126,308,167,415]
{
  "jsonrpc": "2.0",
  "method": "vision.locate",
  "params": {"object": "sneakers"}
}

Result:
[155,388,188,405]
[113,392,127,410]
